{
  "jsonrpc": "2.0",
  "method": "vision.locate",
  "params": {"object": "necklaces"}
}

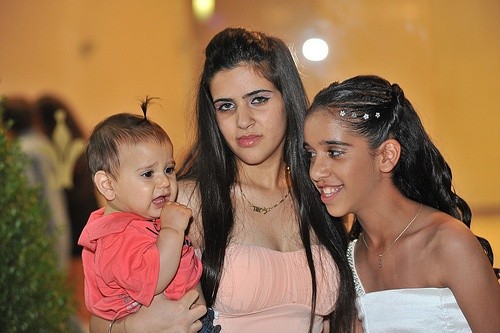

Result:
[237,167,293,214]
[362,203,422,271]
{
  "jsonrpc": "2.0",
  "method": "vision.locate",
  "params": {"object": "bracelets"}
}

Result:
[108,320,115,333]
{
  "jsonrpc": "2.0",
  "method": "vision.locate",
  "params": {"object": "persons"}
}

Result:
[78,94,221,333]
[89,28,356,333]
[0,95,101,333]
[303,75,500,333]
